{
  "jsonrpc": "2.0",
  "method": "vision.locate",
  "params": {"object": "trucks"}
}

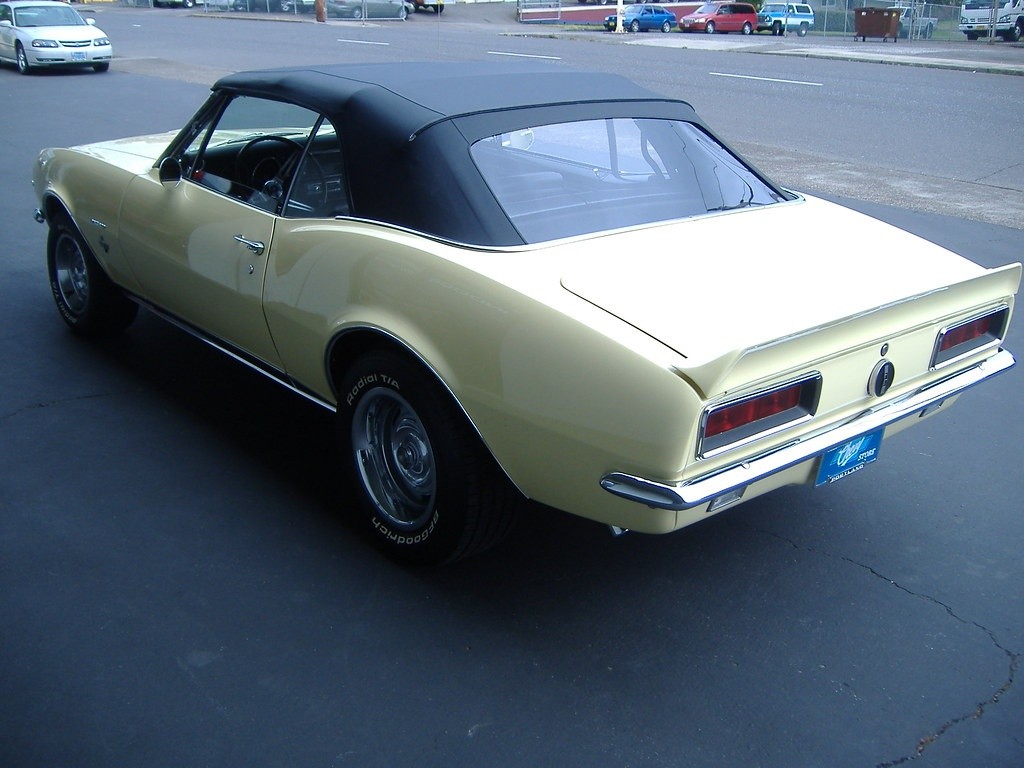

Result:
[885,6,939,39]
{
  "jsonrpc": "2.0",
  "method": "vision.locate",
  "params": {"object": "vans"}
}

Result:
[679,2,758,35]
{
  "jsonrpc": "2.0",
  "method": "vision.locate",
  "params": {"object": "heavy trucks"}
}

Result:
[957,0,1024,44]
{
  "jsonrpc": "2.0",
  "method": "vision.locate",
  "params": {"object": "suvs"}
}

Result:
[754,3,816,37]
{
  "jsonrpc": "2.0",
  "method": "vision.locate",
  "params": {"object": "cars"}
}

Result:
[405,0,456,13]
[578,0,681,7]
[602,3,678,33]
[29,60,1023,564]
[0,0,113,76]
[326,0,415,20]
[152,0,315,15]
